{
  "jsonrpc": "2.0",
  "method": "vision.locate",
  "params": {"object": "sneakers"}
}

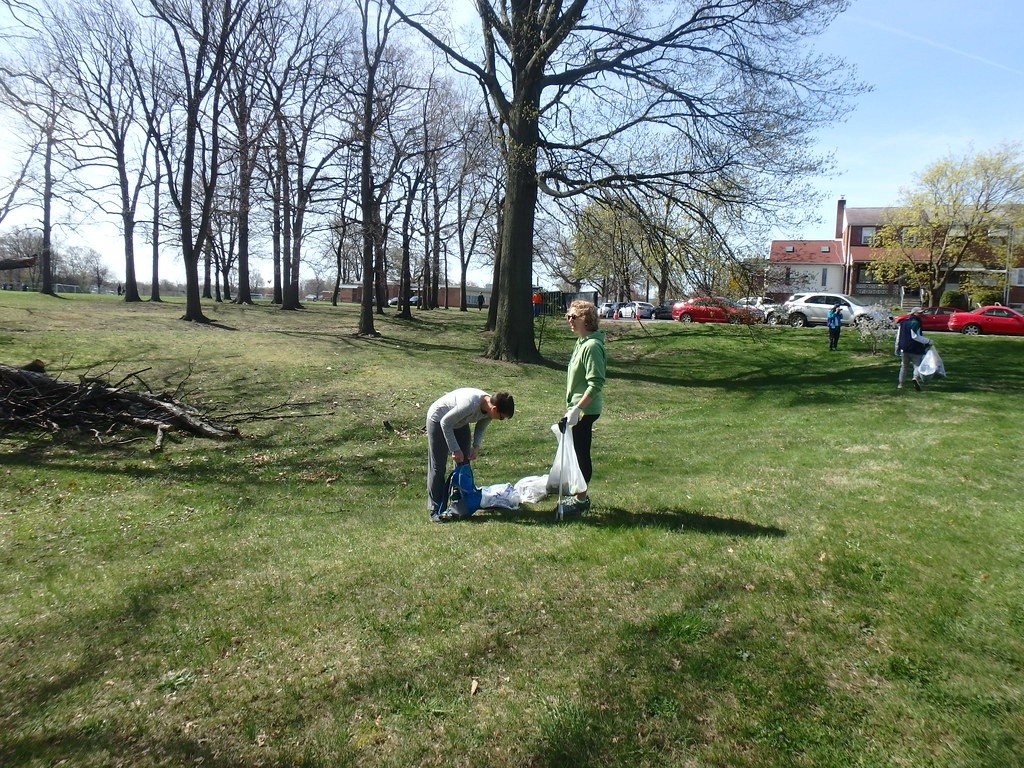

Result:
[556,495,590,513]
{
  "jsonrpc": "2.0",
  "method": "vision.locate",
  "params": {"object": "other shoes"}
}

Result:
[898,383,904,388]
[911,377,920,391]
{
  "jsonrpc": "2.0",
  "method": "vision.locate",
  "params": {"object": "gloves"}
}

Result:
[564,404,582,425]
[928,339,934,345]
[895,348,902,357]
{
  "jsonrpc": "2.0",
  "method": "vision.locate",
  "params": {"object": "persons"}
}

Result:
[426,388,515,522]
[532,291,542,317]
[477,292,485,311]
[894,306,934,391]
[826,303,843,352]
[122,286,126,296]
[554,301,607,515]
[117,283,123,296]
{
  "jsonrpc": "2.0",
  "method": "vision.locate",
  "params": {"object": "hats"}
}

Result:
[909,307,924,315]
[834,303,840,307]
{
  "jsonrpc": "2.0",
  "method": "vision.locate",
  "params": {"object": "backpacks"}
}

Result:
[436,456,482,521]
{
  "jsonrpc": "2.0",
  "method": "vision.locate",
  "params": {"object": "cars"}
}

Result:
[785,293,894,330]
[389,297,403,305]
[618,301,655,319]
[597,303,614,319]
[605,302,628,319]
[410,296,422,306]
[947,306,1024,336]
[895,307,968,331]
[672,297,765,325]
[737,293,806,325]
[650,298,685,320]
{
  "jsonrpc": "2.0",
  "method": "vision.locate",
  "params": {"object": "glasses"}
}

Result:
[568,313,582,320]
[499,412,504,420]
